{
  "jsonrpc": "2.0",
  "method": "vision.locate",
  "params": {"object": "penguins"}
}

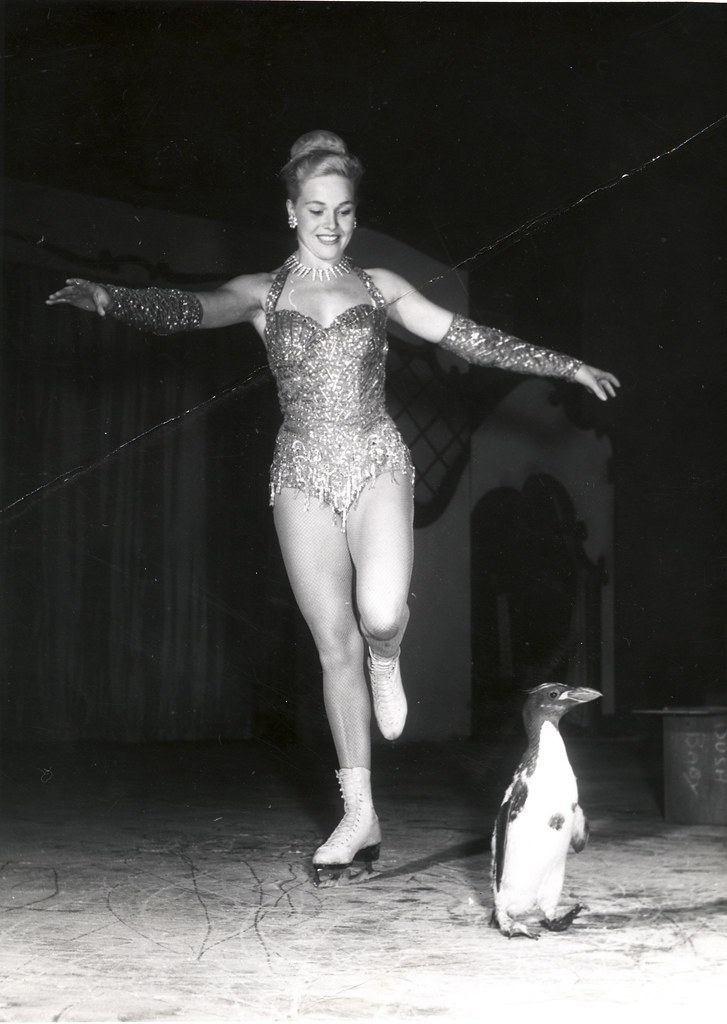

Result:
[488,682,603,940]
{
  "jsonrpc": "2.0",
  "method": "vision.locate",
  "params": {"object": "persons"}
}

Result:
[46,131,621,894]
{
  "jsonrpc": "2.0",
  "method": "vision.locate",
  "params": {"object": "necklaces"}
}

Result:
[285,254,355,284]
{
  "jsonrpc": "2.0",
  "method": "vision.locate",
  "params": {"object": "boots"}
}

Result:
[367,646,407,740]
[311,768,382,887]
